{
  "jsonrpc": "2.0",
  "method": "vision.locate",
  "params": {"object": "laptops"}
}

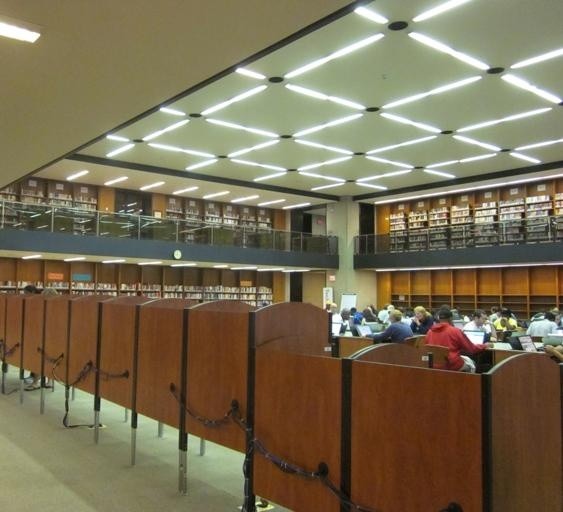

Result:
[330,323,563,354]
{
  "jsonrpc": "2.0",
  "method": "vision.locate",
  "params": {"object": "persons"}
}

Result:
[330,302,562,364]
[420,308,494,372]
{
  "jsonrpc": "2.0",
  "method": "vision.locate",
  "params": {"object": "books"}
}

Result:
[0,187,274,251]
[0,278,273,311]
[384,192,562,251]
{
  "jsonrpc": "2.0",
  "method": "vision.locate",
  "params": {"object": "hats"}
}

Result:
[439,308,452,319]
[390,310,402,319]
[501,308,511,314]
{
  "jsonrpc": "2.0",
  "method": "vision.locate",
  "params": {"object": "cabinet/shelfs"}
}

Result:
[391,294,563,322]
[388,199,563,255]
[0,286,273,309]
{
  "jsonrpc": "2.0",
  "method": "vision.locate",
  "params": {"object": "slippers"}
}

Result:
[25,385,40,390]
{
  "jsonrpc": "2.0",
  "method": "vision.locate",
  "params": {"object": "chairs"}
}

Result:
[405,337,417,345]
[417,344,450,368]
